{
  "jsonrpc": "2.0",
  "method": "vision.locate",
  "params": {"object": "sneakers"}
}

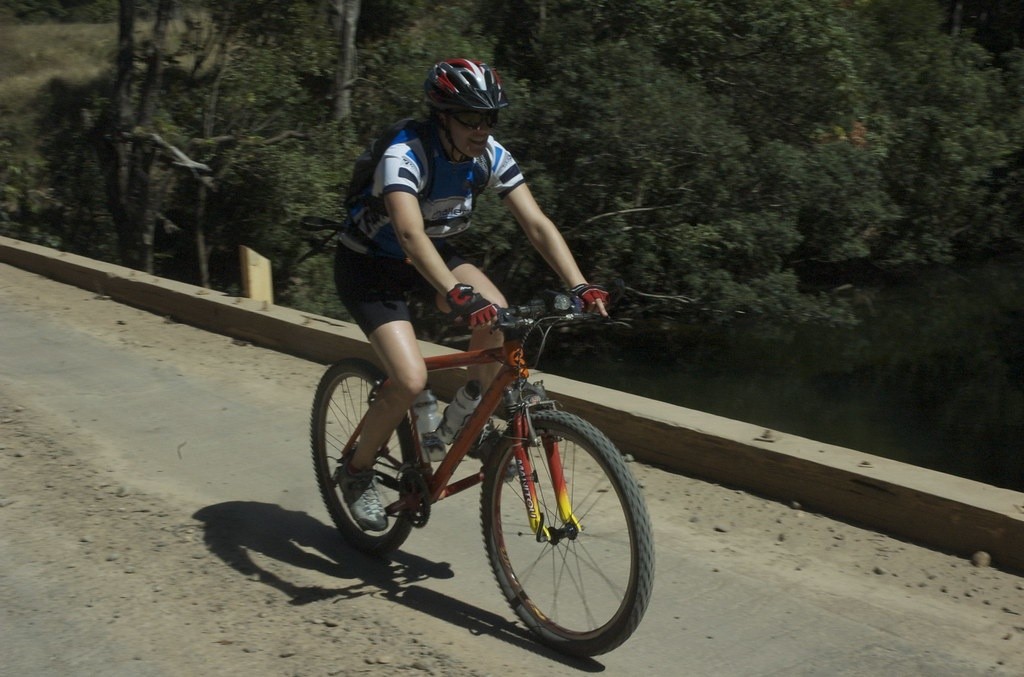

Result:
[335,458,388,532]
[467,416,516,478]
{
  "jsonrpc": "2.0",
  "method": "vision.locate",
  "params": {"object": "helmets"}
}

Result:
[424,57,508,114]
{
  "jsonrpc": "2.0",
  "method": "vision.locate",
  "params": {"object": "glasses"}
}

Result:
[450,111,498,129]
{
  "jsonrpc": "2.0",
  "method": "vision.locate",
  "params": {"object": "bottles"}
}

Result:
[410,381,449,462]
[436,381,483,445]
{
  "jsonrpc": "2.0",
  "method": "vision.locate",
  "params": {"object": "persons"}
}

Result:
[331,56,610,537]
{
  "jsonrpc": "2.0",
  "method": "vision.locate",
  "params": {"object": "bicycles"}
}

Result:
[308,290,657,659]
